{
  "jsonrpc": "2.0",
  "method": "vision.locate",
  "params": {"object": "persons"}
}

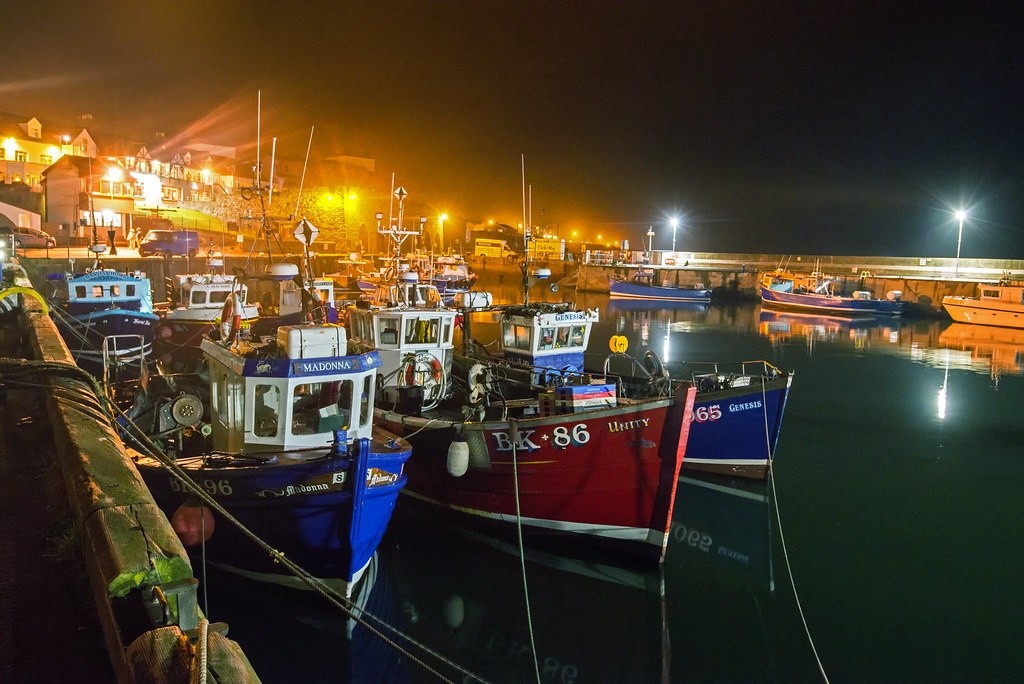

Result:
[126,229,136,249]
[136,227,143,248]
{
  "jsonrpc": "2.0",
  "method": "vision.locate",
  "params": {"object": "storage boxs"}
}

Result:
[276,322,347,359]
[491,396,540,419]
[538,383,617,418]
[206,259,224,269]
[89,244,108,253]
[399,272,418,284]
[264,262,299,280]
[531,269,551,279]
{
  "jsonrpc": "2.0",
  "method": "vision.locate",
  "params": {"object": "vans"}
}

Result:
[138,230,200,258]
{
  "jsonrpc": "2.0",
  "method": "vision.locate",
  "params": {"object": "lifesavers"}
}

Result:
[406,353,442,389]
[307,290,327,327]
[383,266,396,283]
[219,294,242,347]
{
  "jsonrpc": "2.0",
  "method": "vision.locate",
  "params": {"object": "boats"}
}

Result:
[755,252,909,318]
[483,154,795,490]
[605,272,719,302]
[938,321,1024,392]
[608,295,711,314]
[756,304,909,359]
[43,187,163,362]
[941,268,1024,330]
[152,226,259,351]
[334,261,700,566]
[103,250,414,610]
[237,88,495,343]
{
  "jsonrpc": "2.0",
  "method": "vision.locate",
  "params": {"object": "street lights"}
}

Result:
[955,210,968,279]
[439,214,448,255]
[670,218,679,258]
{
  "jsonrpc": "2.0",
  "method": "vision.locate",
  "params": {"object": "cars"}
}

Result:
[0,225,57,250]
